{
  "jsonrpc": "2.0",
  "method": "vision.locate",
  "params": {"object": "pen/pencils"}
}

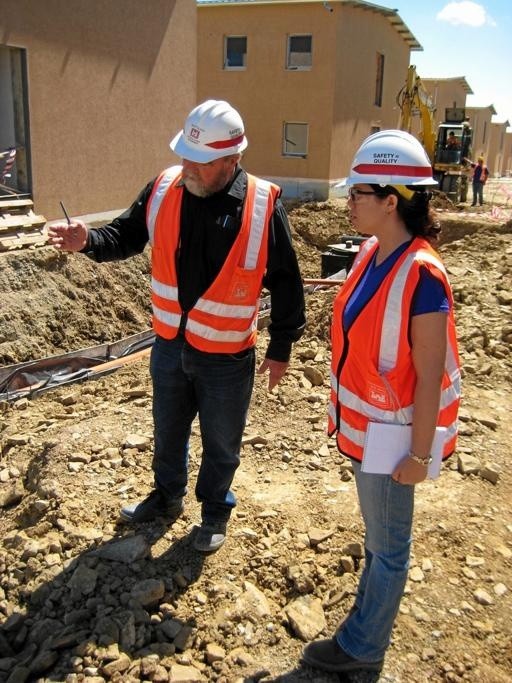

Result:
[59,200,78,238]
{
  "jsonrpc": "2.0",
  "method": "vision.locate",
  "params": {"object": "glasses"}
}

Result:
[349,186,376,200]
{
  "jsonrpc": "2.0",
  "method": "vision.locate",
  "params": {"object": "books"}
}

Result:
[360,421,448,480]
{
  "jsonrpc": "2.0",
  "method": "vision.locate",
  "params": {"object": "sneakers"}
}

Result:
[120,497,182,521]
[300,607,385,671]
[195,521,227,552]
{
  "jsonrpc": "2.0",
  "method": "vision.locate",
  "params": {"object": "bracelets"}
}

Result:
[408,451,434,467]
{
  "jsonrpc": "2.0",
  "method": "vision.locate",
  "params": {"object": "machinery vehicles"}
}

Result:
[396,65,474,203]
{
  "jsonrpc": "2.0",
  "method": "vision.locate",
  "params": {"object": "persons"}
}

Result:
[445,132,461,151]
[299,128,464,669]
[46,98,308,555]
[461,155,489,208]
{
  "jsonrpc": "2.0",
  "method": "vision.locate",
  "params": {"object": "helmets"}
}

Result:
[170,98,249,164]
[345,128,439,190]
